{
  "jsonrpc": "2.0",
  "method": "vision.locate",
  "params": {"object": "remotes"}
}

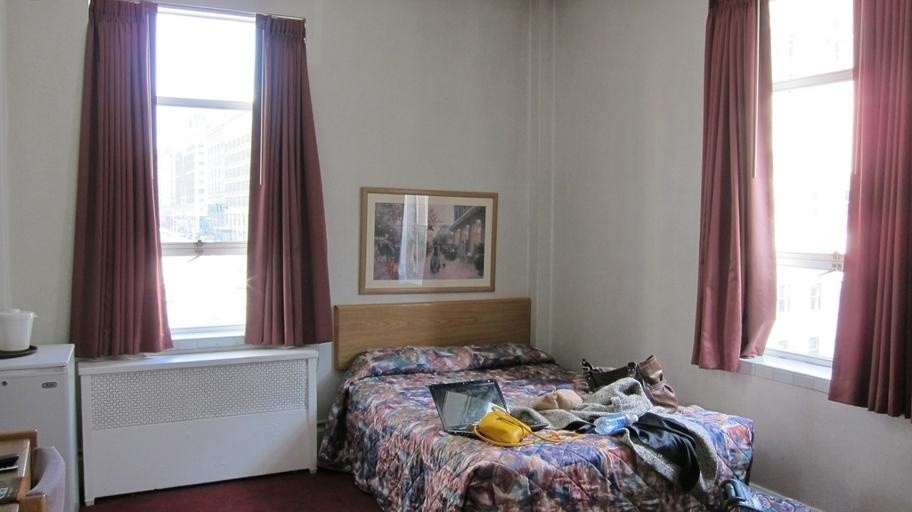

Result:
[0,453,19,468]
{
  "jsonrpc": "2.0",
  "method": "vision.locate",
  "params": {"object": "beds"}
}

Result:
[333,297,755,511]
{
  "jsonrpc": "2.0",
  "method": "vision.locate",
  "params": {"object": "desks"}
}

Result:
[0,429,38,512]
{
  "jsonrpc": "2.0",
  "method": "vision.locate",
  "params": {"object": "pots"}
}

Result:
[0,307,38,350]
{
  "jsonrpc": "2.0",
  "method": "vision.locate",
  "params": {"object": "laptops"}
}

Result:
[429,379,549,438]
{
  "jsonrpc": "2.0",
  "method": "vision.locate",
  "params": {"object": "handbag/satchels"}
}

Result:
[577,354,681,418]
[471,403,584,447]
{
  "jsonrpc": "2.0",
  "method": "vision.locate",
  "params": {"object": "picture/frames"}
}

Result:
[357,186,498,294]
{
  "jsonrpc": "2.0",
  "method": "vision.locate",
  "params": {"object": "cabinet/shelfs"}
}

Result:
[0,343,79,512]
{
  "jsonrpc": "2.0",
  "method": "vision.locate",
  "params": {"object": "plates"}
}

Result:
[0,344,40,360]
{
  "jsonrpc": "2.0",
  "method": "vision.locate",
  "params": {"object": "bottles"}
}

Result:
[593,413,639,436]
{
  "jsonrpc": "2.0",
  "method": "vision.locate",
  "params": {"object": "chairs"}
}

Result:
[20,447,65,512]
